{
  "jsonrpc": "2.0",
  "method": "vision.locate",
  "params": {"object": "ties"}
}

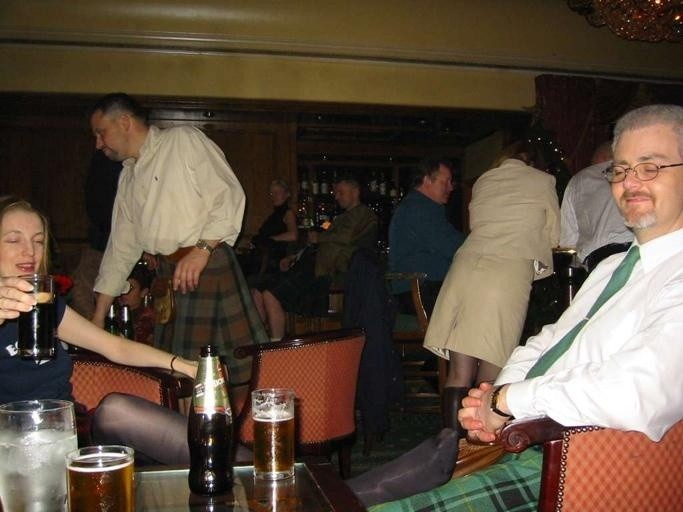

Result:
[521,243,641,383]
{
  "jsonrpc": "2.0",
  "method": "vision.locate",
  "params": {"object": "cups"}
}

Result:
[0,398,78,512]
[64,443,135,512]
[14,272,56,362]
[250,387,295,480]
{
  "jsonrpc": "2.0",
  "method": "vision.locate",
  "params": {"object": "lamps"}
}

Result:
[566,0,682,42]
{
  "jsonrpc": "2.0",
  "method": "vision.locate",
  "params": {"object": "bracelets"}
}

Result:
[170,355,179,370]
[488,382,511,417]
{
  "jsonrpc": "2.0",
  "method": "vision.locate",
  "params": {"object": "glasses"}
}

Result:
[600,161,682,183]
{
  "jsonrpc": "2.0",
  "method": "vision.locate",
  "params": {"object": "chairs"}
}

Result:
[229,327,369,480]
[497,410,680,511]
[380,267,447,431]
[289,288,354,334]
[67,351,195,416]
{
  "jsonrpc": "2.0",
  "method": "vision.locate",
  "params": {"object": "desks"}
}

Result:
[123,457,365,512]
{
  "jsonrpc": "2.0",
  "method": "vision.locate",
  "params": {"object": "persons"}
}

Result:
[369,102,683,512]
[557,141,635,272]
[424,131,562,429]
[88,92,273,388]
[0,195,457,505]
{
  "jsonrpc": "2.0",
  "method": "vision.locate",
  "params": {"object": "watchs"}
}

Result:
[196,239,216,254]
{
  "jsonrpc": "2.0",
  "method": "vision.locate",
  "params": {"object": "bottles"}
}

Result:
[186,343,235,496]
[365,179,399,216]
[299,173,339,232]
[105,303,134,340]
